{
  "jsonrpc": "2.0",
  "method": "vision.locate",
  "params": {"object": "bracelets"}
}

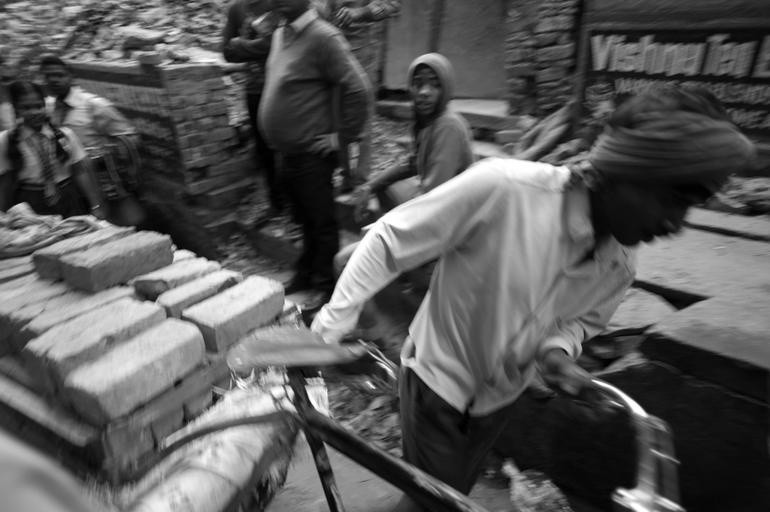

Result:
[90,204,102,212]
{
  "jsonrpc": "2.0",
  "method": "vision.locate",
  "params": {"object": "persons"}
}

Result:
[258,0,374,314]
[308,0,403,98]
[0,81,107,221]
[331,52,476,329]
[40,57,146,226]
[310,83,756,511]
[222,1,307,231]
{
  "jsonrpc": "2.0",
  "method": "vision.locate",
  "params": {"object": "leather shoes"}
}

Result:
[299,287,332,313]
[283,270,312,296]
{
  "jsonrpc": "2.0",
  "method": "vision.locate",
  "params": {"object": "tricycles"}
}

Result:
[2,267,687,512]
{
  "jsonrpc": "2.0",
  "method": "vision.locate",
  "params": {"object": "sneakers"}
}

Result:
[269,212,305,240]
